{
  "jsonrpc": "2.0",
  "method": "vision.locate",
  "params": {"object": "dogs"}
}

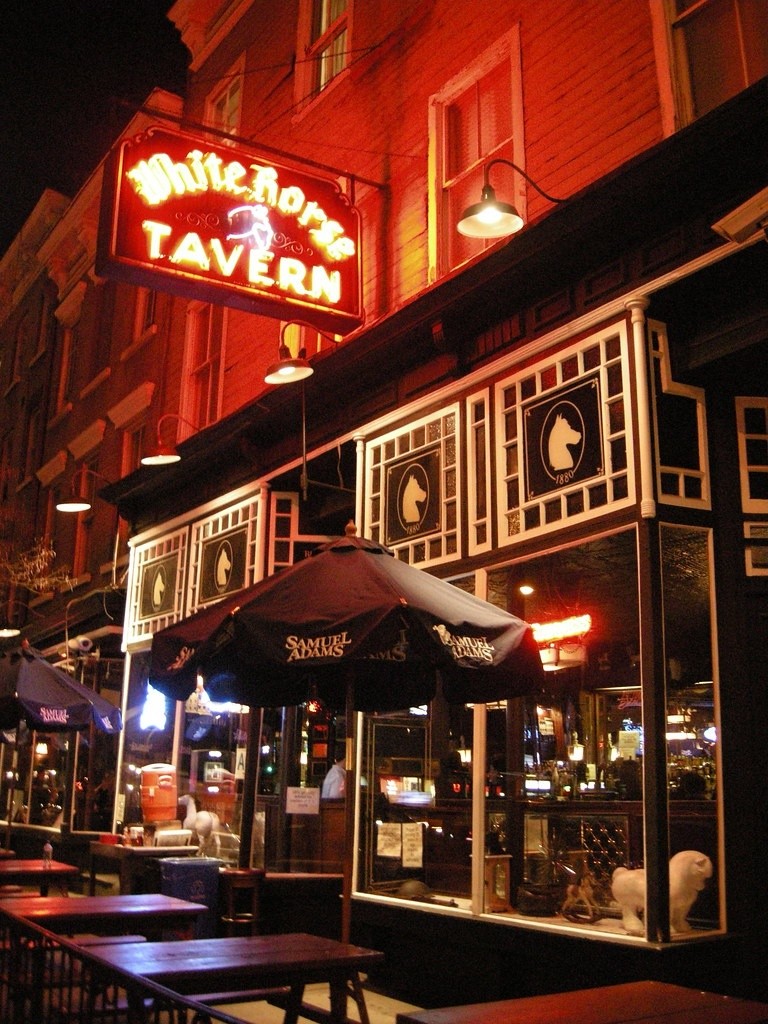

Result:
[612,850,714,936]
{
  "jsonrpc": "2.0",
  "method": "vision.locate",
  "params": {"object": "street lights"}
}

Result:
[566,731,586,799]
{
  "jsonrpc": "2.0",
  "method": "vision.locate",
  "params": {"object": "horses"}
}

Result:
[178,794,221,857]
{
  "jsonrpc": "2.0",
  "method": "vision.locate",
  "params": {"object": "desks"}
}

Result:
[0,846,768,1024]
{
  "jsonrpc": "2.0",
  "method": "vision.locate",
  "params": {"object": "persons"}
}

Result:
[669,772,707,801]
[617,760,643,802]
[32,766,52,803]
[322,744,347,800]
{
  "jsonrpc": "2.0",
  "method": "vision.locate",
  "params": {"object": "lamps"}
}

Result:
[264,319,340,384]
[55,469,112,513]
[456,157,563,238]
[141,413,200,466]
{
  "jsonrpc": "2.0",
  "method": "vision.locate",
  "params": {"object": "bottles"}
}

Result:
[122,827,130,846]
[42,840,52,869]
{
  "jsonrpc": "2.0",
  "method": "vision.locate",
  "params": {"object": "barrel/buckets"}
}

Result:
[141,763,177,824]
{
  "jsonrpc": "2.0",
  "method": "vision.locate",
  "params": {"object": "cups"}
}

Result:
[130,826,143,847]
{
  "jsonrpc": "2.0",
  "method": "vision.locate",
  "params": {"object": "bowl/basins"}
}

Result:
[98,833,120,845]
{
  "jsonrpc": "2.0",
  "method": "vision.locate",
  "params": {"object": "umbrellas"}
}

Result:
[149,520,544,943]
[0,638,123,825]
